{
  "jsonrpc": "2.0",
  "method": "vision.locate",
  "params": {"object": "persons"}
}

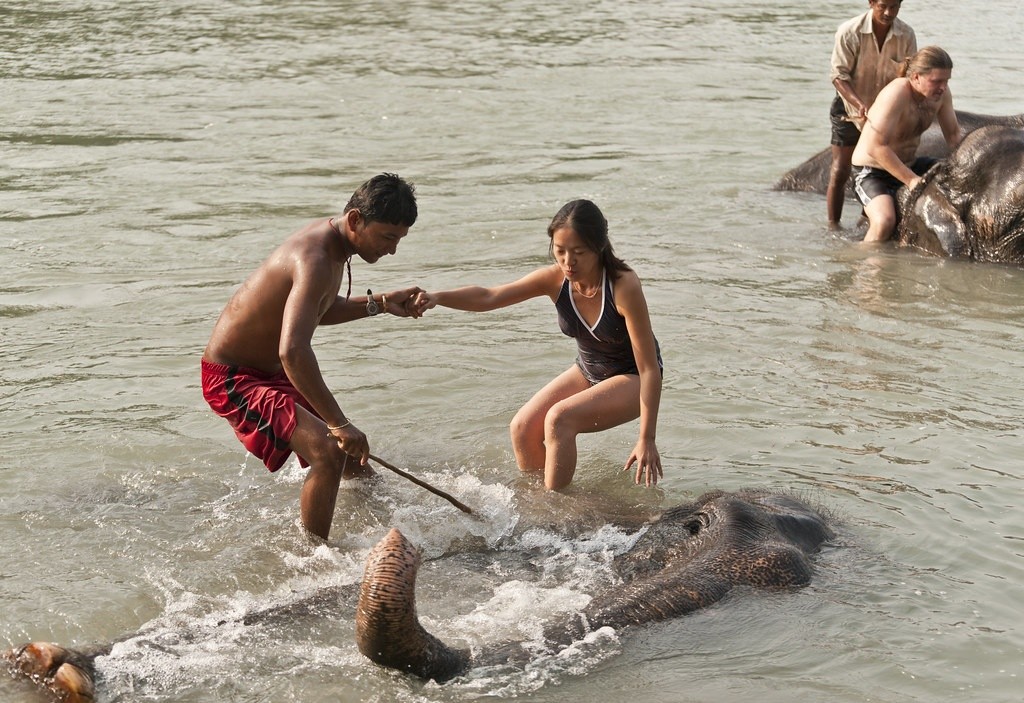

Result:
[405,199,664,488]
[851,45,961,242]
[201,173,425,540]
[827,0,917,230]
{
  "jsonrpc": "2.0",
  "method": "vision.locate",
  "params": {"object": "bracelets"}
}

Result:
[327,417,351,430]
[381,293,387,314]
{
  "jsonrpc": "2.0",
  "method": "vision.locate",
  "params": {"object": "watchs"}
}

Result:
[365,289,378,316]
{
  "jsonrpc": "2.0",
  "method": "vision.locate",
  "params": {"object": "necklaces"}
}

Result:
[329,217,352,304]
[573,272,603,298]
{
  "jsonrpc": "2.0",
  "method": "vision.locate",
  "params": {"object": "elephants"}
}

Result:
[770,107,1024,268]
[204,486,836,684]
